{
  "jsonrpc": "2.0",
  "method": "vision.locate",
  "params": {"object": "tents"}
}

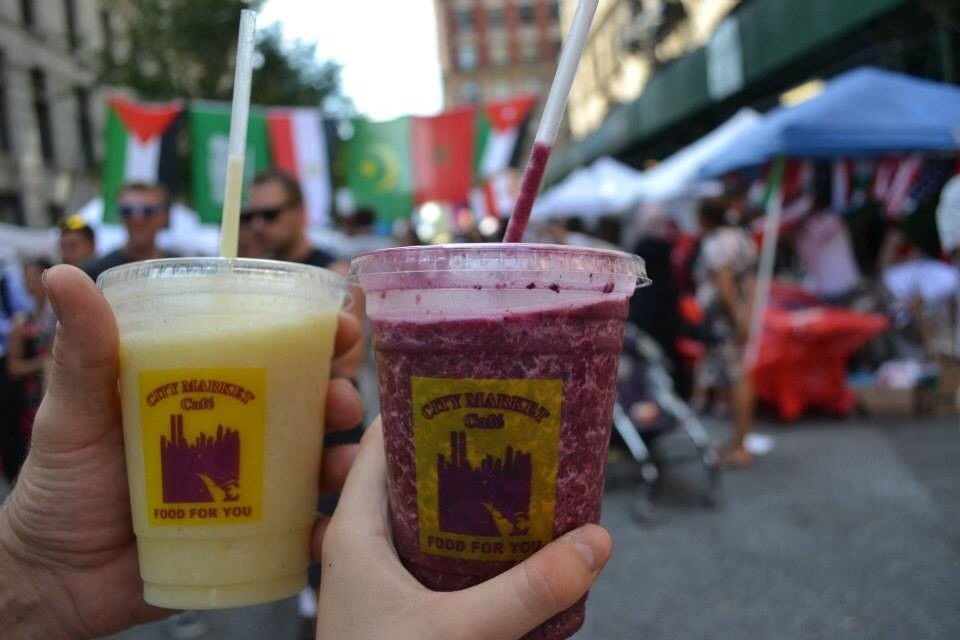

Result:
[526,67,960,465]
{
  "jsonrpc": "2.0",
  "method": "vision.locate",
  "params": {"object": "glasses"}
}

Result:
[121,205,163,220]
[240,202,294,224]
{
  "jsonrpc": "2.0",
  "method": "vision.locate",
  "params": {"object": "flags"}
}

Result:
[103,94,540,228]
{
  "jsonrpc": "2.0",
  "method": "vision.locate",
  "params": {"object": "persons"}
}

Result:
[0,158,864,483]
[0,263,613,640]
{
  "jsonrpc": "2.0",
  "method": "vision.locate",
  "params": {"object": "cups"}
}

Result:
[97,258,347,611]
[352,243,653,640]
